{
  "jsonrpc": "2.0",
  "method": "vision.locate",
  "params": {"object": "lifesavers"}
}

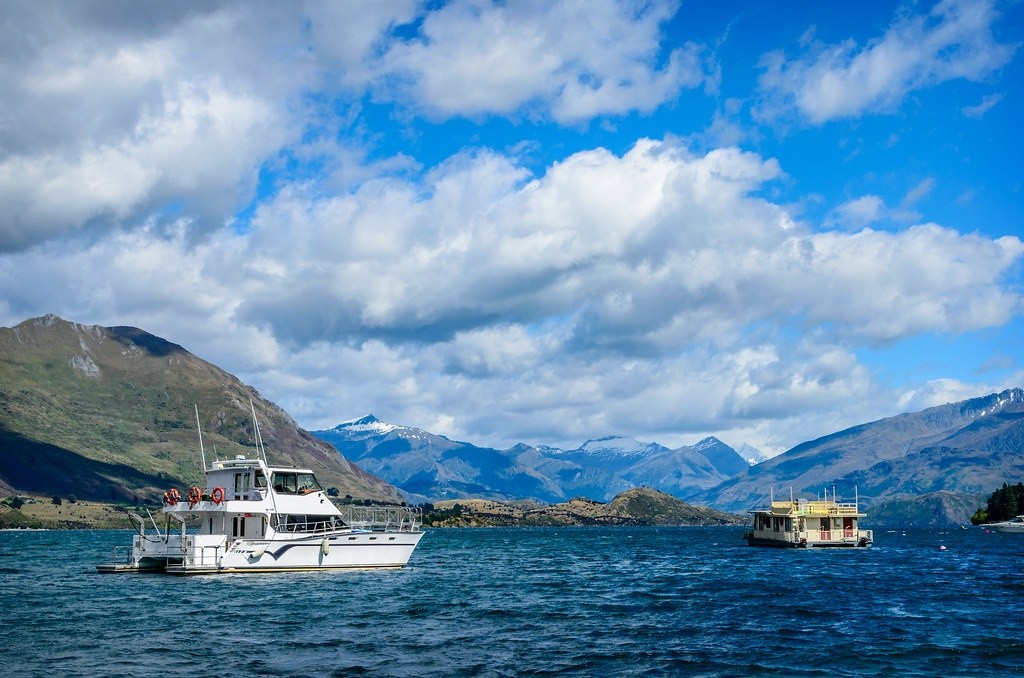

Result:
[189,486,202,503]
[212,487,224,503]
[167,489,179,505]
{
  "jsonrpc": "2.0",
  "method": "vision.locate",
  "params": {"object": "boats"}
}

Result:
[747,486,874,548]
[95,399,426,574]
[978,515,1024,533]
